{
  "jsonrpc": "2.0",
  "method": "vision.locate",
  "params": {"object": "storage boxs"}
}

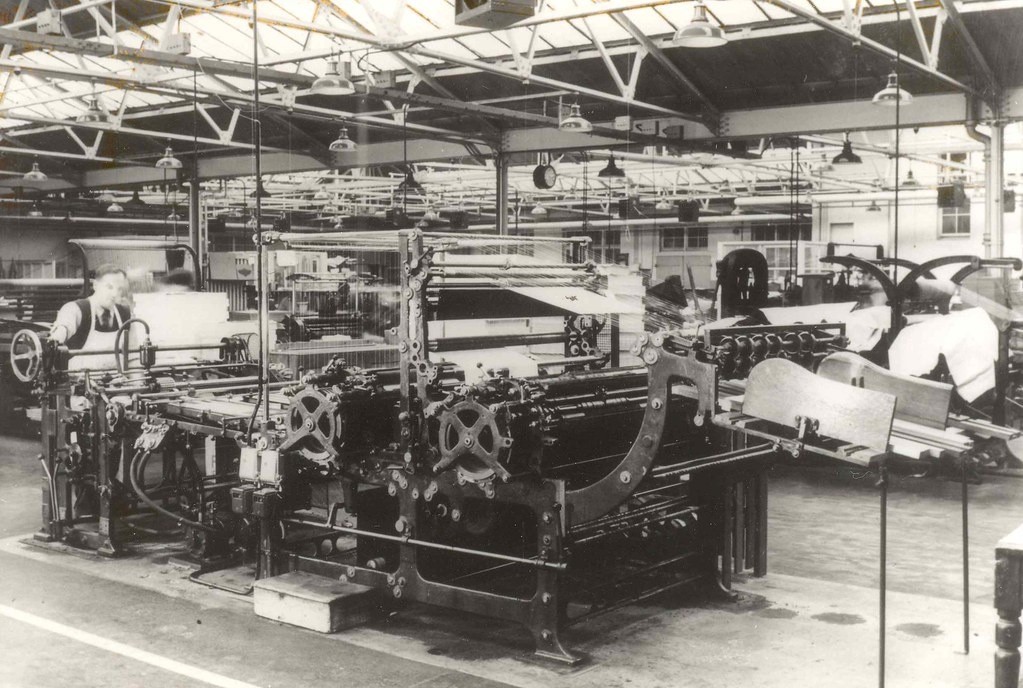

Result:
[252,570,378,634]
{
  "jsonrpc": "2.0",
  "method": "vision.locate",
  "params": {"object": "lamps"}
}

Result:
[20,0,922,213]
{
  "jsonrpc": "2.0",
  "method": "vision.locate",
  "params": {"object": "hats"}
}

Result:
[160,268,192,286]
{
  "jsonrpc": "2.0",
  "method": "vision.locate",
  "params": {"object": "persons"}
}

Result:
[160,268,195,293]
[48,266,130,517]
